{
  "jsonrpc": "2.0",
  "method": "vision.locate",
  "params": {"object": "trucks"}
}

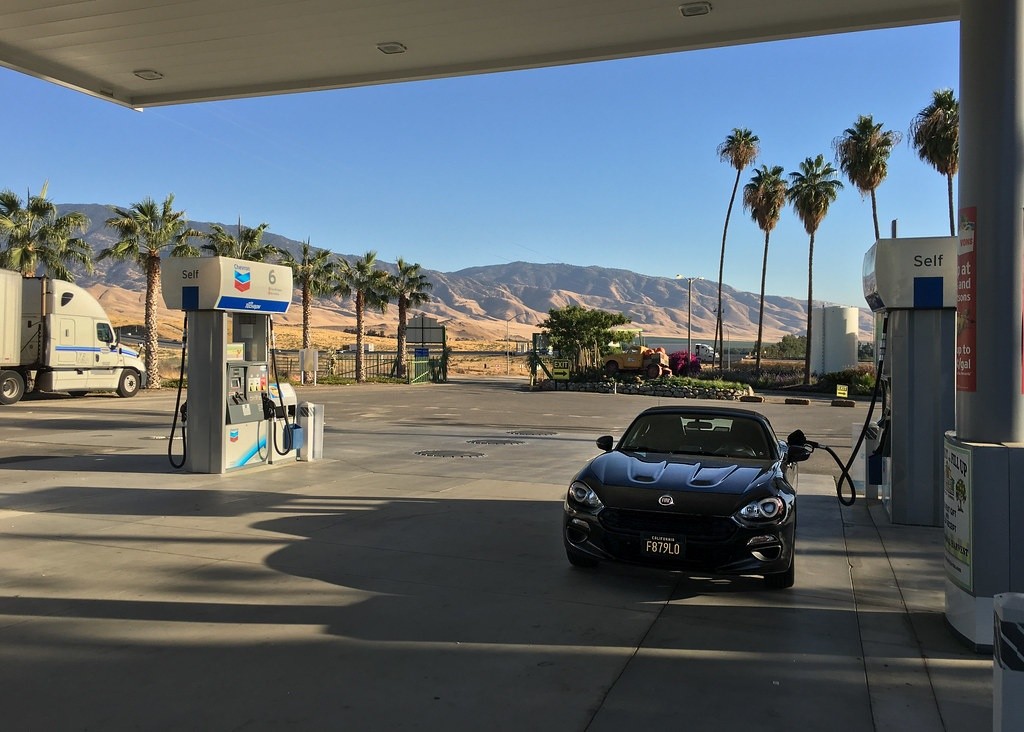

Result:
[0,269,148,406]
[694,343,719,363]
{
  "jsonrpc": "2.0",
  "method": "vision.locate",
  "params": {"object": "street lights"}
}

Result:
[506,312,531,375]
[676,274,704,366]
[722,323,731,371]
[712,307,725,367]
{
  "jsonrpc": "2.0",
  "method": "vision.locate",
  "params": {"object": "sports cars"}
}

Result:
[561,405,812,589]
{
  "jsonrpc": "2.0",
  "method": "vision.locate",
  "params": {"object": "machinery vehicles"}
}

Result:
[601,345,673,380]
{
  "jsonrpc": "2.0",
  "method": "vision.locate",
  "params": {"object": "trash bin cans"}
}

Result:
[268,383,299,462]
